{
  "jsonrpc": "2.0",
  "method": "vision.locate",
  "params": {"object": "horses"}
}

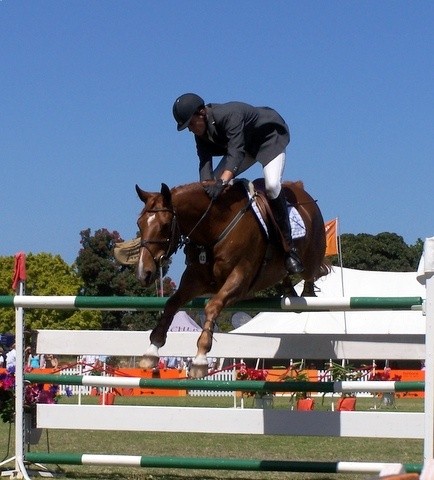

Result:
[135,178,334,377]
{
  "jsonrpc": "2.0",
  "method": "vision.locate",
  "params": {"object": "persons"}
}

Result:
[173,93,305,274]
[0,343,247,379]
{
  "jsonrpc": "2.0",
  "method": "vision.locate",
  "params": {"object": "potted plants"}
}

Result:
[91,355,402,411]
[13,413,42,445]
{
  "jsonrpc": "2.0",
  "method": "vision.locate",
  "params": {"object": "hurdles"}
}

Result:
[0,237,434,480]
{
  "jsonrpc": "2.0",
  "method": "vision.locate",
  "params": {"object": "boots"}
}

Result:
[267,189,304,274]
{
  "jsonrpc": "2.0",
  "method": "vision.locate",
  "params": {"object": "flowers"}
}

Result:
[0,365,74,422]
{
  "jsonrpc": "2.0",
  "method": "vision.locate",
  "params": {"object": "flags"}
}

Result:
[324,217,338,257]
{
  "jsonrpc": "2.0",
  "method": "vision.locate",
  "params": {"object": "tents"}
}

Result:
[229,264,426,370]
[146,310,203,332]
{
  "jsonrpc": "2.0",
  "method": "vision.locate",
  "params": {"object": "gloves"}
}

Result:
[204,179,228,201]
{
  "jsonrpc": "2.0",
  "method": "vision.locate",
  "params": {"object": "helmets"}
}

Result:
[172,93,206,132]
